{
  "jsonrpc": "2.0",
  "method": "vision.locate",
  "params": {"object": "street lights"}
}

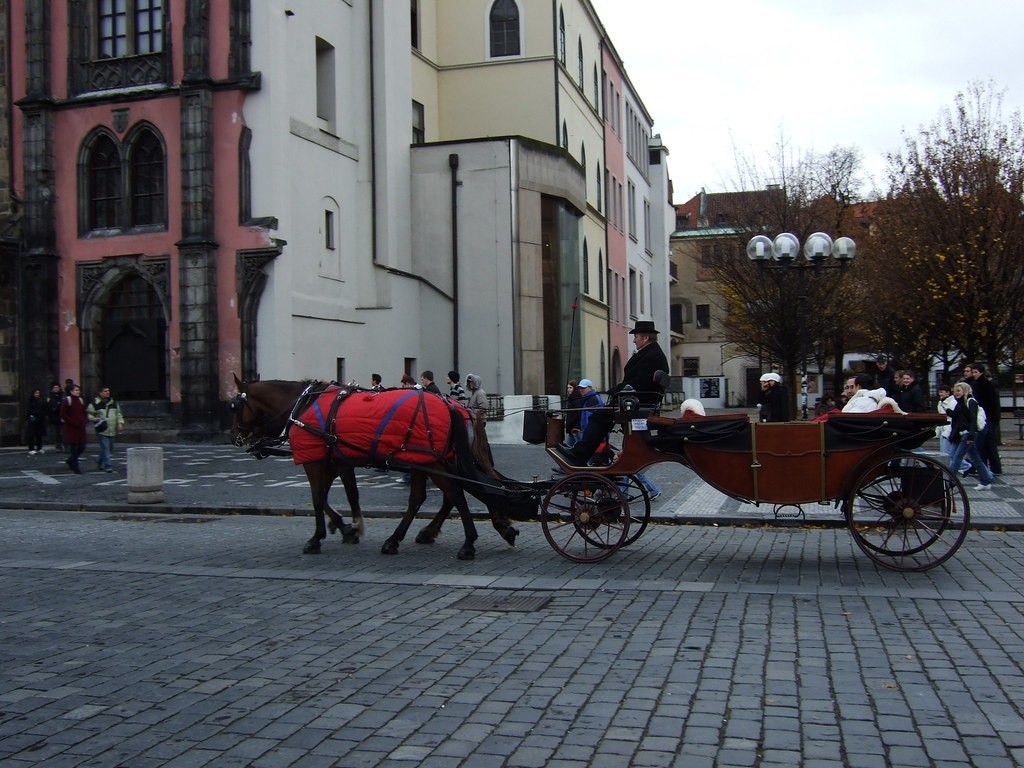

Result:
[747,231,858,419]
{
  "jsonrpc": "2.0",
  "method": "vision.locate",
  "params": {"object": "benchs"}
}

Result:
[614,370,671,423]
[812,405,892,423]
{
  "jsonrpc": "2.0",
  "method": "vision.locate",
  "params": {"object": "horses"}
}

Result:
[229,371,521,561]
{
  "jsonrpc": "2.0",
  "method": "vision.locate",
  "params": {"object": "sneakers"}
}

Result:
[974,484,992,490]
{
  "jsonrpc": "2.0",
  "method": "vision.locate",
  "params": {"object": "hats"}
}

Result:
[448,371,459,383]
[629,321,660,334]
[876,355,886,363]
[577,379,592,387]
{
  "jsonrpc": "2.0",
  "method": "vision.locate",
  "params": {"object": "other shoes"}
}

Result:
[30,450,36,455]
[650,492,660,500]
[105,465,112,472]
[37,450,45,454]
[558,443,579,465]
[71,465,81,474]
[97,459,105,470]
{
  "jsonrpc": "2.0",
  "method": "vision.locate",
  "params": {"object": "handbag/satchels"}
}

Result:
[967,398,986,431]
[94,419,108,432]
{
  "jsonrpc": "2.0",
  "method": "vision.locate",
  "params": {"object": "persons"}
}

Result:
[876,354,897,397]
[397,376,425,484]
[892,370,930,467]
[422,371,441,396]
[615,468,661,502]
[445,371,466,407]
[64,379,75,396]
[812,373,886,418]
[945,382,994,490]
[45,382,66,448]
[557,320,669,468]
[935,385,973,477]
[86,386,126,473]
[60,385,89,474]
[759,373,792,423]
[25,389,46,455]
[464,374,488,427]
[574,378,610,443]
[958,363,1003,477]
[371,374,389,472]
[552,379,582,475]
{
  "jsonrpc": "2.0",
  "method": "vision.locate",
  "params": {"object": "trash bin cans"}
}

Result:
[127,446,164,504]
[907,451,950,501]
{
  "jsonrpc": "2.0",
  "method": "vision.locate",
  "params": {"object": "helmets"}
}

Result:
[760,373,780,382]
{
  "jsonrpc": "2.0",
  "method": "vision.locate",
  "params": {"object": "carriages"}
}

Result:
[225,370,972,573]
